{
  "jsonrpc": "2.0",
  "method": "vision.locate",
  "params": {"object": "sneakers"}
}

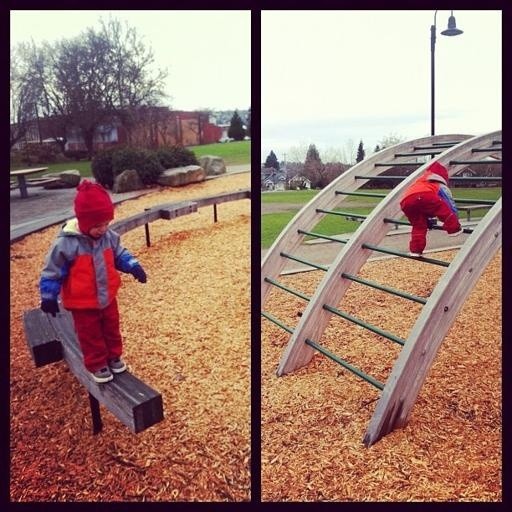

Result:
[91,367,114,384]
[108,356,127,374]
[449,227,464,237]
[409,250,422,257]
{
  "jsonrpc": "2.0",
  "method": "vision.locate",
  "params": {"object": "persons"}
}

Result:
[400,162,463,257]
[41,179,147,384]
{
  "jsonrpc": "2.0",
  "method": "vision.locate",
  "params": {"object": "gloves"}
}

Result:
[130,263,147,283]
[428,218,437,230]
[40,297,60,317]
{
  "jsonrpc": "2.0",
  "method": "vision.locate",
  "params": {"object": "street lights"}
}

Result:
[426,8,466,167]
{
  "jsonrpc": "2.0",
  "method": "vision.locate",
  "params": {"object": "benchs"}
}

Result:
[452,204,492,221]
[10,165,63,200]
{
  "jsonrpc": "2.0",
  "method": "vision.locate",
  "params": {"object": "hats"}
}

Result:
[426,162,448,183]
[75,178,115,235]
